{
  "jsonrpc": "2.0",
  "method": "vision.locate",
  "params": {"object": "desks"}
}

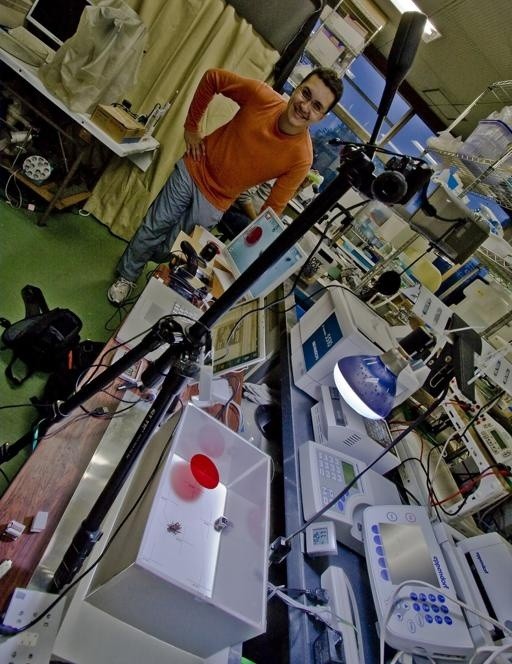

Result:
[0,45,161,227]
[0,261,243,625]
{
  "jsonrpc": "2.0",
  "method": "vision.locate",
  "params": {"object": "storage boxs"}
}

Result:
[458,119,511,185]
[407,180,492,265]
[89,103,146,143]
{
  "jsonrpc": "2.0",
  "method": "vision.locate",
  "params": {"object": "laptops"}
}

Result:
[114,277,267,380]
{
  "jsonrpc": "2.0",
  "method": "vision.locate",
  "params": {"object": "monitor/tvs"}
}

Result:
[25,0,96,48]
[213,207,308,309]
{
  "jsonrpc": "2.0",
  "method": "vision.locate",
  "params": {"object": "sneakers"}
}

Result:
[106,270,138,309]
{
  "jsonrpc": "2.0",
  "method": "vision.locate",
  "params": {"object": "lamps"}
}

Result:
[270,326,449,563]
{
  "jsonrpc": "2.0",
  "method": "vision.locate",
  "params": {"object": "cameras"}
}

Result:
[375,146,434,209]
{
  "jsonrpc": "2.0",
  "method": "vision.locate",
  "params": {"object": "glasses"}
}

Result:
[296,85,330,116]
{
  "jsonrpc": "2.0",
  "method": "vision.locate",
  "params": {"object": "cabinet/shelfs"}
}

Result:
[325,79,512,292]
[286,0,384,91]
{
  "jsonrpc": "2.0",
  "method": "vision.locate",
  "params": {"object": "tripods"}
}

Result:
[1,163,403,591]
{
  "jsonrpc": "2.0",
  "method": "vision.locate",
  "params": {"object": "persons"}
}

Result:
[221,168,320,237]
[106,66,345,305]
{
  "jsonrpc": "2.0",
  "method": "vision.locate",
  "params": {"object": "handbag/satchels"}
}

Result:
[0,284,83,386]
